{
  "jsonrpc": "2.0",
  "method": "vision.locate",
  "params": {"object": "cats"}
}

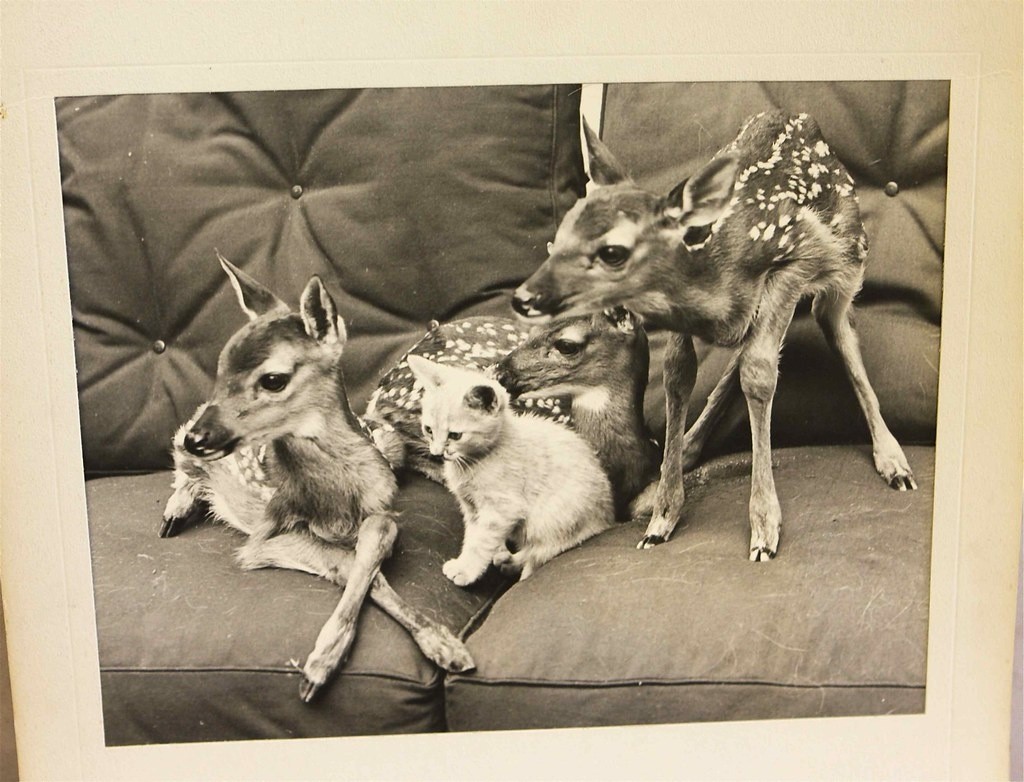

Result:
[407,352,615,588]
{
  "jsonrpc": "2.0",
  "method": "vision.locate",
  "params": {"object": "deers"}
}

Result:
[363,306,662,521]
[160,246,476,702]
[510,110,917,563]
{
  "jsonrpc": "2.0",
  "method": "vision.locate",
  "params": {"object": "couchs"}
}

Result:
[55,77,951,746]
